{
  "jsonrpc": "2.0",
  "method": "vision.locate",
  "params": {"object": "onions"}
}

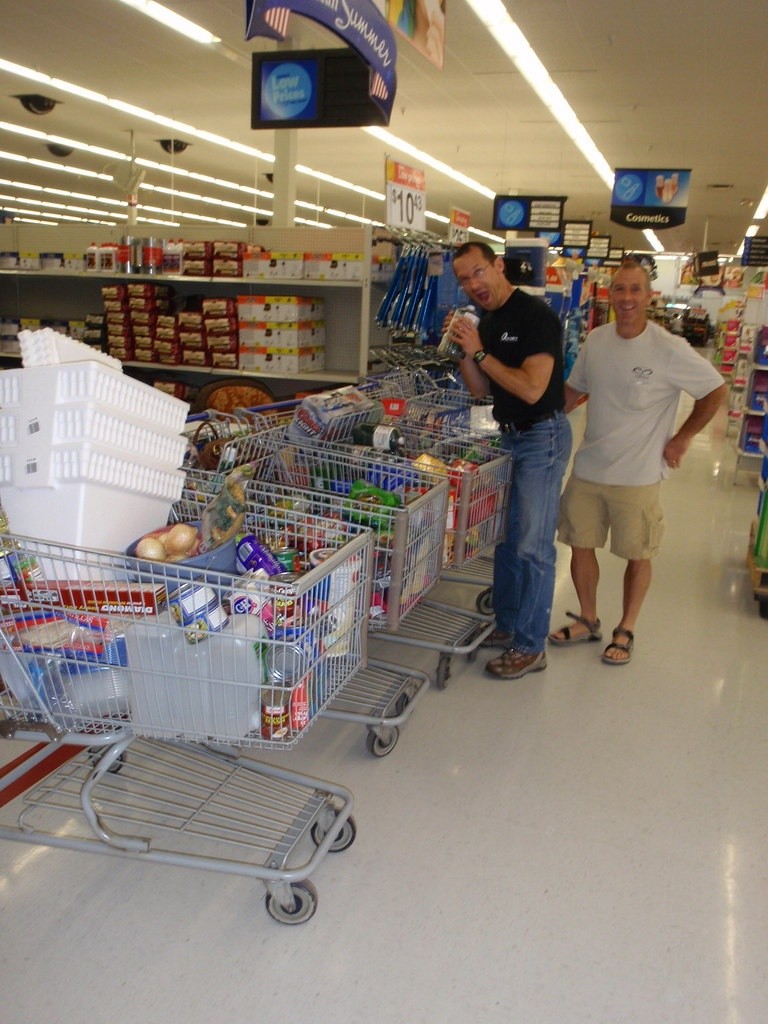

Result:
[136,522,202,563]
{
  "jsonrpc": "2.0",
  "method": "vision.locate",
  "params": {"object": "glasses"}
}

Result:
[458,261,492,285]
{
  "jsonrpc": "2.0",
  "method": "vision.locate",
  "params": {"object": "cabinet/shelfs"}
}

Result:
[719,326,768,487]
[0,223,374,385]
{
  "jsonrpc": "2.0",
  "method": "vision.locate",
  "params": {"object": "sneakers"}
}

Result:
[485,646,548,678]
[470,625,514,647]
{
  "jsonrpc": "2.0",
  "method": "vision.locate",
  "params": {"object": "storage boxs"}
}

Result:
[682,311,709,347]
[504,237,549,287]
[0,241,384,617]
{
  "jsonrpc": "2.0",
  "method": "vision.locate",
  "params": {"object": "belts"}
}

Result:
[498,412,553,435]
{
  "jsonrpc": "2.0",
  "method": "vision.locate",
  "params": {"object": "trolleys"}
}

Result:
[1,359,514,926]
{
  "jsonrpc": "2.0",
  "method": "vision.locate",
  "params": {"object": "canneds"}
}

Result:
[163,533,363,742]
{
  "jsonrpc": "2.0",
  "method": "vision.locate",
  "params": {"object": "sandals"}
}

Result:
[600,627,635,664]
[547,611,603,647]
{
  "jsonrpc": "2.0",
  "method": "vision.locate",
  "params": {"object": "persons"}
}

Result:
[549,263,725,665]
[442,242,571,677]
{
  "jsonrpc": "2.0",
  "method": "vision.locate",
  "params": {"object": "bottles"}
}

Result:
[85,242,119,273]
[350,423,405,451]
[172,239,185,275]
[162,239,176,275]
[437,305,480,362]
[218,447,237,474]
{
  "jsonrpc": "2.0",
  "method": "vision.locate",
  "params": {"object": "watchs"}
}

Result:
[473,350,488,363]
